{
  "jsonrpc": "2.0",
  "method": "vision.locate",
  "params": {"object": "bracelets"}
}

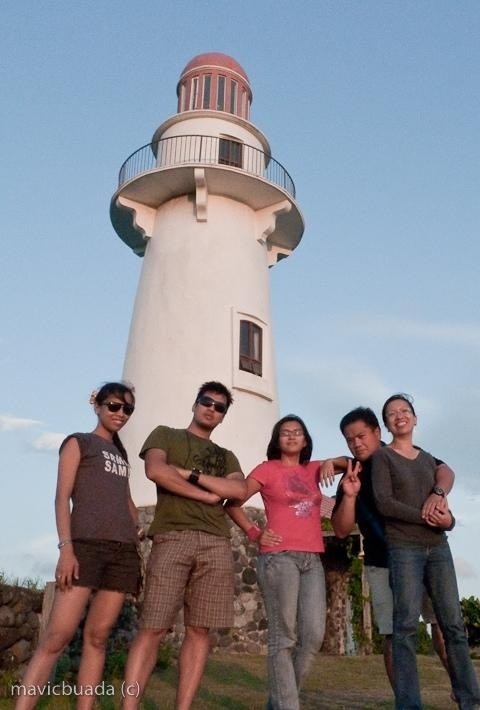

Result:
[58,539,73,549]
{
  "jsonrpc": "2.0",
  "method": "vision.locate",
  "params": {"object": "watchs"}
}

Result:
[186,467,202,485]
[428,486,445,498]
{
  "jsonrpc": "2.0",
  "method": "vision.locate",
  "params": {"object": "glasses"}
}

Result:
[385,407,411,418]
[197,395,226,414]
[277,429,306,437]
[103,401,134,415]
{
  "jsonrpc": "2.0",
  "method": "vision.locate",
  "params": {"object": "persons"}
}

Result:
[121,379,248,709]
[371,394,479,710]
[330,405,455,709]
[14,379,145,710]
[223,412,352,709]
